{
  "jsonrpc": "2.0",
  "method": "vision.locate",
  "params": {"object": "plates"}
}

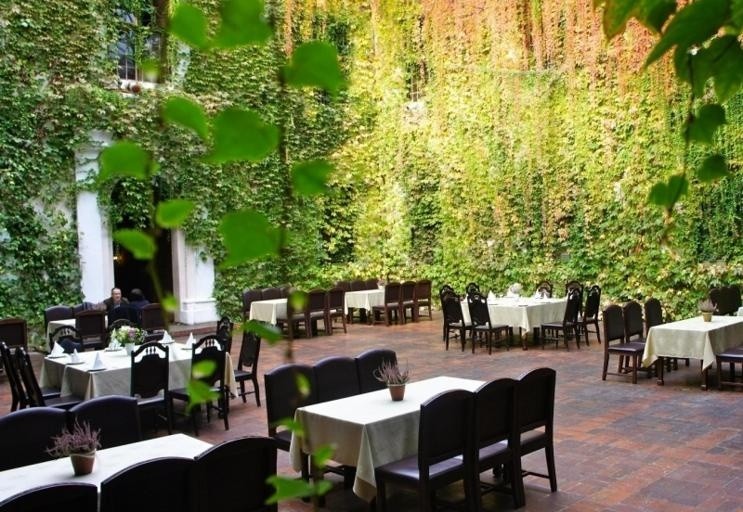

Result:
[66,362,85,365]
[182,346,193,350]
[105,347,122,352]
[48,354,67,358]
[89,368,106,372]
[462,292,550,306]
[158,339,175,343]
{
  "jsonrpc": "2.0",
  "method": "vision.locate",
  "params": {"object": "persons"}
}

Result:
[100,287,129,318]
[125,287,150,327]
